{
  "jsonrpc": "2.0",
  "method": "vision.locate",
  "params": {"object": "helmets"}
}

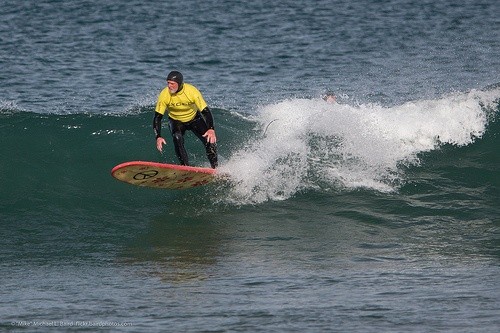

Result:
[167,70,183,84]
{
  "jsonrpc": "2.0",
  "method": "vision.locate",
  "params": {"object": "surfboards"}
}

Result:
[111,161,218,191]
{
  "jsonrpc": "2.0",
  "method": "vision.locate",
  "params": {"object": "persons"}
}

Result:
[152,70,219,169]
